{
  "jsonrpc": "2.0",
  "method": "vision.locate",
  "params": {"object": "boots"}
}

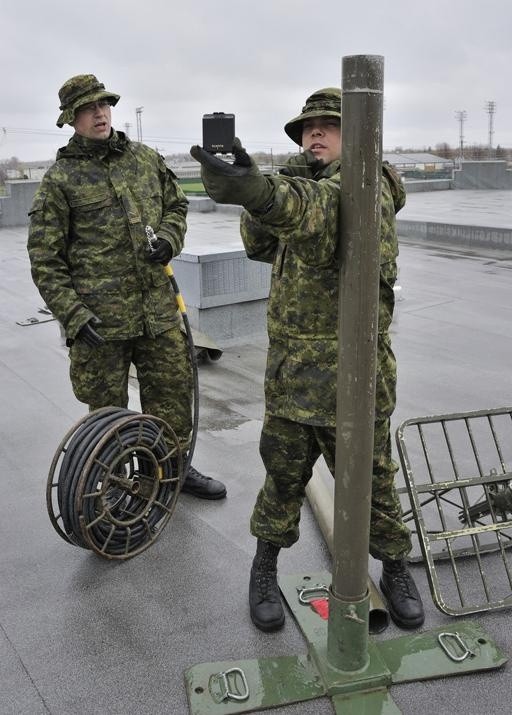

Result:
[247,538,286,634]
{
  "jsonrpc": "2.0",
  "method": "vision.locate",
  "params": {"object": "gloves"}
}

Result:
[149,238,174,266]
[79,316,105,348]
[189,137,276,214]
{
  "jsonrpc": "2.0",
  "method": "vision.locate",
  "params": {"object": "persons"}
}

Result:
[26,73,229,502]
[190,87,427,634]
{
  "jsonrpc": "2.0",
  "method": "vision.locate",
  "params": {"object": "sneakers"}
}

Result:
[171,464,227,501]
[378,558,426,630]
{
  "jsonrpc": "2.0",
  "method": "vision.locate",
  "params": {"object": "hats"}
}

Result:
[56,73,122,129]
[283,87,345,147]
